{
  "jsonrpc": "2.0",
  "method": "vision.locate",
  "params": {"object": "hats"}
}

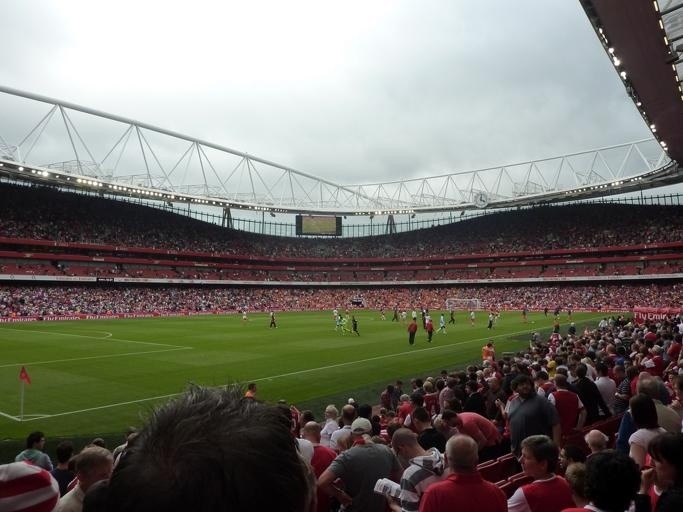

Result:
[0,461,61,512]
[350,416,372,435]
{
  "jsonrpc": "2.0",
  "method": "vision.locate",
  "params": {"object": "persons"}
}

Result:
[1,187,682,512]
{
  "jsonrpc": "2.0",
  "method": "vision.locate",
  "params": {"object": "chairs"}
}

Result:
[478,410,626,495]
